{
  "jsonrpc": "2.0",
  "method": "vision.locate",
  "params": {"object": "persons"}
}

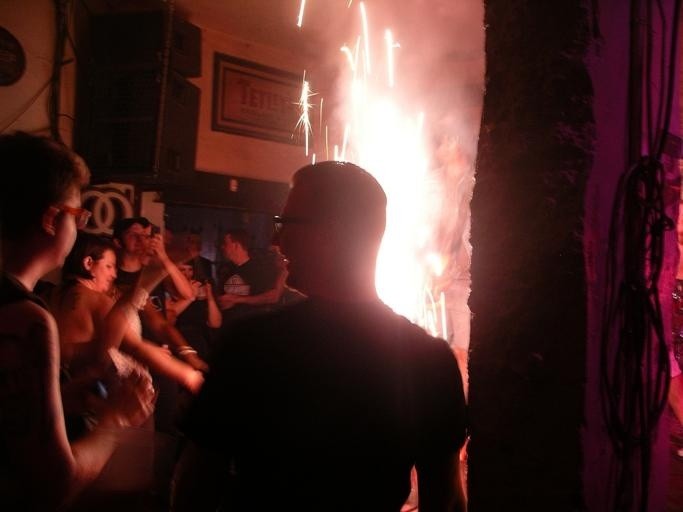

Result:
[0,127,158,511]
[167,158,477,511]
[35,214,289,447]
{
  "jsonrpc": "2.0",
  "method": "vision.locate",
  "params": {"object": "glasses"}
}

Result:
[271,215,319,225]
[50,201,91,230]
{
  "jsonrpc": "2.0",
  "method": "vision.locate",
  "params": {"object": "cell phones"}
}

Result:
[151,226,160,239]
